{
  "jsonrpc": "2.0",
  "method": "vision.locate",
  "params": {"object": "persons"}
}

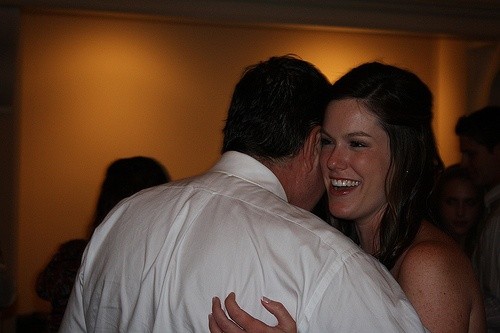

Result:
[57,53,500,332]
[33,155,171,333]
